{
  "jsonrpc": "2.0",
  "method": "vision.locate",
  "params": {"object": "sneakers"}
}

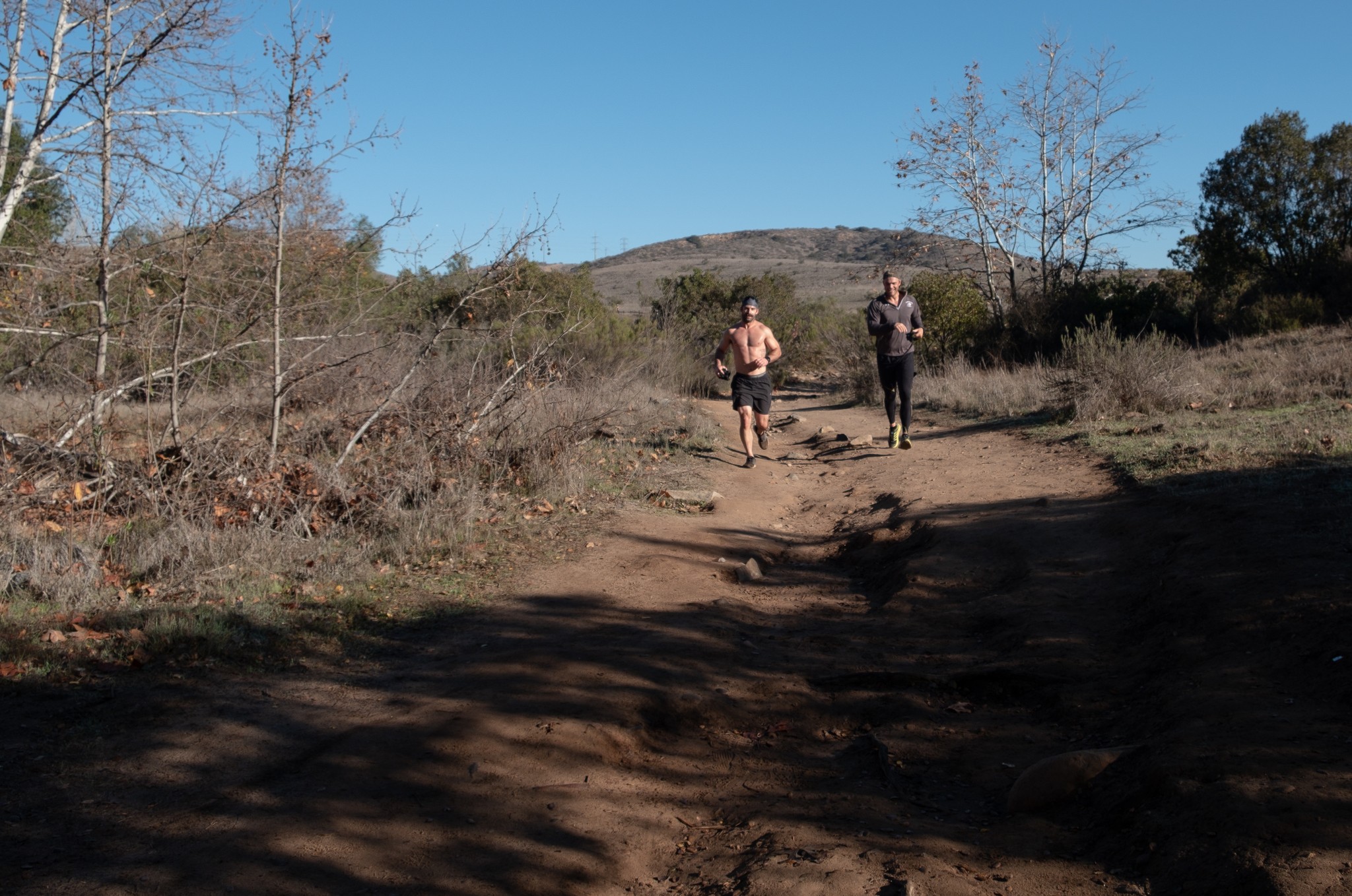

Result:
[887,421,900,448]
[898,435,911,450]
[753,424,768,449]
[742,456,756,469]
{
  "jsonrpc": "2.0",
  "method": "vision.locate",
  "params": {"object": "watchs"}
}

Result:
[764,357,770,363]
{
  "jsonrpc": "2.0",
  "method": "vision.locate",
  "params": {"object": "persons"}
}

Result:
[714,295,782,469]
[867,269,924,449]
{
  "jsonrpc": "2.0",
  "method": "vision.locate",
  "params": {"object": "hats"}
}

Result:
[883,269,900,279]
